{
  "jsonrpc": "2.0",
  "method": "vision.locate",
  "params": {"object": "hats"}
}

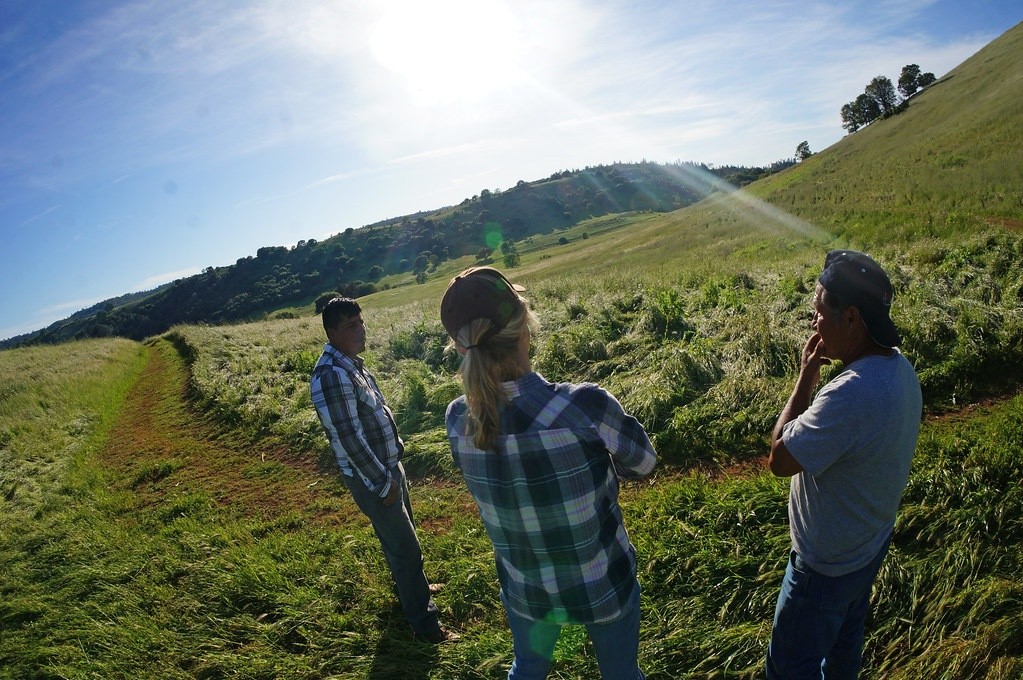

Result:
[818,250,900,348]
[440,267,526,345]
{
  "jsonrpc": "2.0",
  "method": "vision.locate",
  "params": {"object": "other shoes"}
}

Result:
[429,583,445,594]
[415,626,461,643]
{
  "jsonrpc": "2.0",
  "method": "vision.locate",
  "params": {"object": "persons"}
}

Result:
[441,267,657,680]
[310,298,461,648]
[766,250,922,680]
